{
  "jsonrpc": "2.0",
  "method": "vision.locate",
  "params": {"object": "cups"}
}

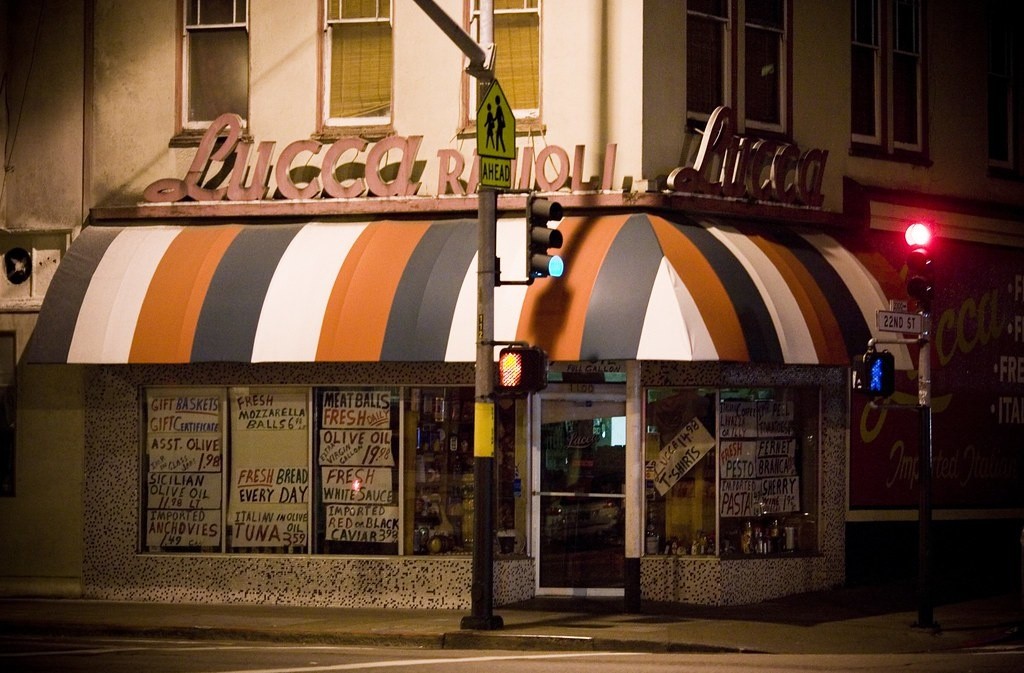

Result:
[498,535,515,553]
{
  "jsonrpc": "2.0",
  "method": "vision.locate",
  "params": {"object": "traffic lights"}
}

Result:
[939,262,973,304]
[907,217,936,300]
[525,193,566,279]
[856,351,899,399]
[501,347,538,392]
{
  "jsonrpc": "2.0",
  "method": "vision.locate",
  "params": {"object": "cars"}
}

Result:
[540,469,619,543]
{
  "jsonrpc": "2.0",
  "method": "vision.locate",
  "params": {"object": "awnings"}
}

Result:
[19,214,917,372]
